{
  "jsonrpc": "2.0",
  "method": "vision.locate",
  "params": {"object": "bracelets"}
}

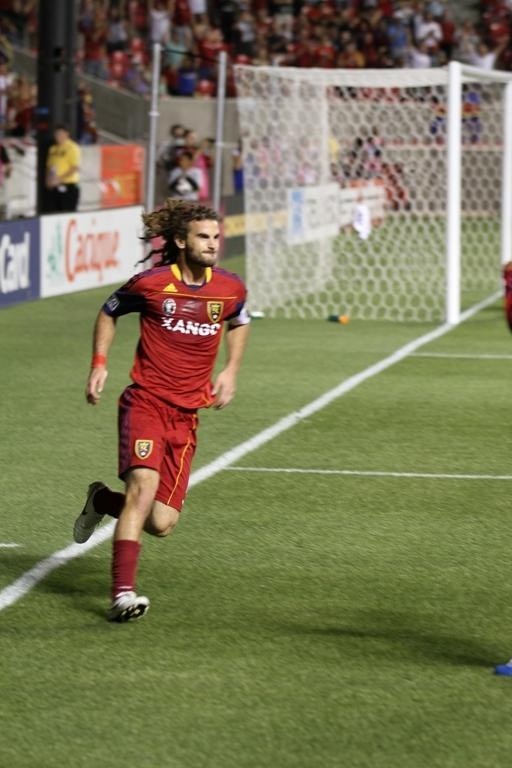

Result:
[90,352,107,367]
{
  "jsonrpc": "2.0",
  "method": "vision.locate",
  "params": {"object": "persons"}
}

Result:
[1,0,511,221]
[61,196,253,630]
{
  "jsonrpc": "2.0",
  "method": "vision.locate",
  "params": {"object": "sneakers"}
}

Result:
[111,590,150,621]
[74,483,110,543]
